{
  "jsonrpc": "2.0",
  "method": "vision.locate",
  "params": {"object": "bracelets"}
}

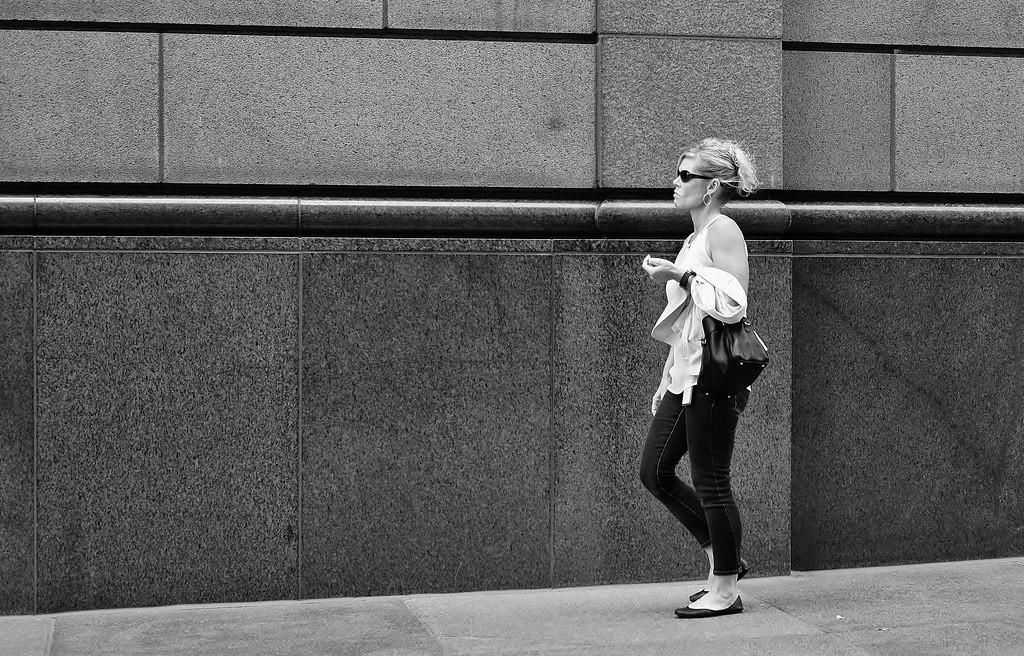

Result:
[679,269,697,289]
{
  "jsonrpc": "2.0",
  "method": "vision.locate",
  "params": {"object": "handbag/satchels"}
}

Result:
[697,313,770,400]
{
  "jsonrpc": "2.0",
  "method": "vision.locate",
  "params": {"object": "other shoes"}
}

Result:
[689,559,751,601]
[675,596,744,617]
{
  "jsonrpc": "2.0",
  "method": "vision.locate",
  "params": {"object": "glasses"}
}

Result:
[677,168,725,187]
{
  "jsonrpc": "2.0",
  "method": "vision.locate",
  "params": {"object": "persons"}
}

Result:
[640,136,770,617]
